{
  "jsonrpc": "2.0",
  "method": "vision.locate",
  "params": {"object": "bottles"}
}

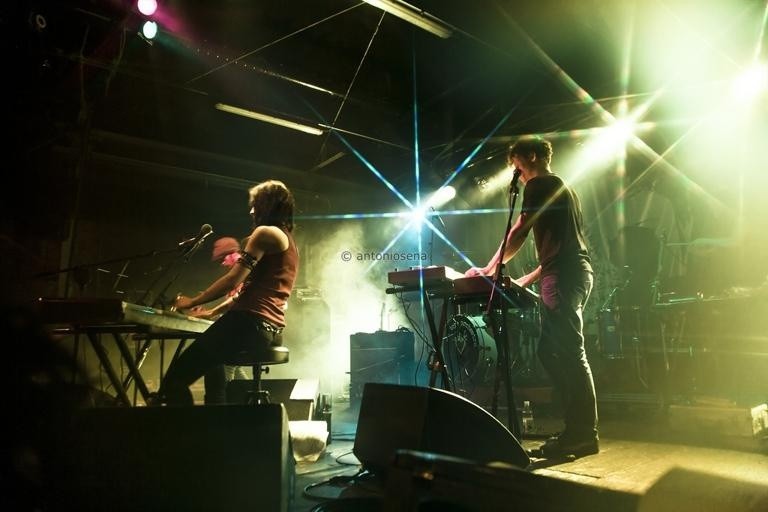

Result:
[522,401,535,435]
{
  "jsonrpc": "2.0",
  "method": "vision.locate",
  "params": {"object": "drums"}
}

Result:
[442,313,514,386]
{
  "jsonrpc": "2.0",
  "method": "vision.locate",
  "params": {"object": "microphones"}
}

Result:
[180,222,213,257]
[429,203,447,232]
[510,166,522,188]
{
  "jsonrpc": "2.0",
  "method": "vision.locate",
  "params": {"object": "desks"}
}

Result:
[386,283,537,443]
[49,322,199,407]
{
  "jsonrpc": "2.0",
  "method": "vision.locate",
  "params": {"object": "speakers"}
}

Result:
[64,404,283,512]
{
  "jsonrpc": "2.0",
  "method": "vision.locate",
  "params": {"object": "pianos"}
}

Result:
[452,266,540,309]
[40,295,215,338]
[388,264,466,295]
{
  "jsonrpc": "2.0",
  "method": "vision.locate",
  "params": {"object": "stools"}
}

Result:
[223,348,288,408]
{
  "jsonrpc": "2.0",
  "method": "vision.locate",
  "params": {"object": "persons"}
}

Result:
[463,135,600,460]
[154,181,302,409]
[192,236,249,381]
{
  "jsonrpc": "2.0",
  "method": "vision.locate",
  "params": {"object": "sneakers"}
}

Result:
[539,430,600,457]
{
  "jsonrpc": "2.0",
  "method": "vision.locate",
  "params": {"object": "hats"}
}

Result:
[210,236,241,263]
